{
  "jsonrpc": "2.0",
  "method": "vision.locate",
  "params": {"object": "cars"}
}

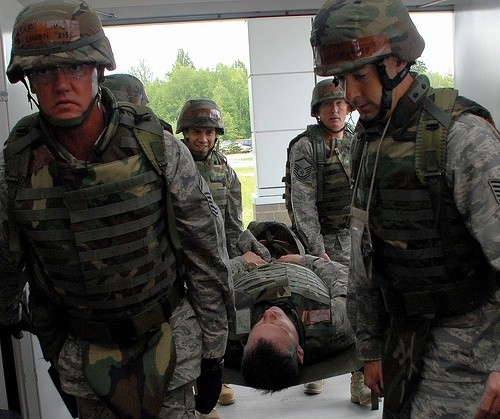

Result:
[221,138,252,153]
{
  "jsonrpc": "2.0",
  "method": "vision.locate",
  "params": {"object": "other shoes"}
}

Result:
[350,373,382,406]
[195,406,219,419]
[217,383,235,404]
[304,378,324,393]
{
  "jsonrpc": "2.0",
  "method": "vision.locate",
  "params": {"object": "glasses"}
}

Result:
[27,64,89,83]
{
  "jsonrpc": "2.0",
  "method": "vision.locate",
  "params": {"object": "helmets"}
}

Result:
[311,79,356,117]
[101,74,149,106]
[176,99,224,135]
[310,0,426,77]
[6,0,116,84]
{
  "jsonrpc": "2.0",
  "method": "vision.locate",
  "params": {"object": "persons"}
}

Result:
[310,0,500,419]
[176,100,244,419]
[223,221,357,395]
[0,0,235,419]
[282,79,382,419]
